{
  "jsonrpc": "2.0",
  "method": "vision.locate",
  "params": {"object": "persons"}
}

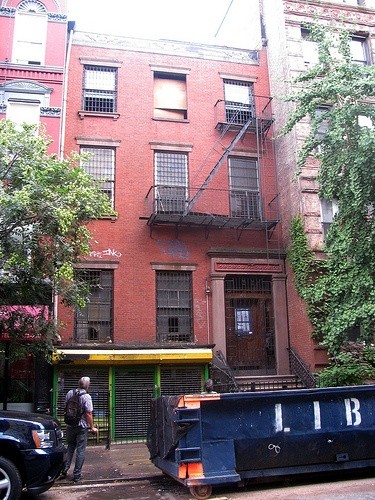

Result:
[201,379,217,394]
[60,377,98,481]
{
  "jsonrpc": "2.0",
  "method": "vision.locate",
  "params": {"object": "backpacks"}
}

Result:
[64,388,90,426]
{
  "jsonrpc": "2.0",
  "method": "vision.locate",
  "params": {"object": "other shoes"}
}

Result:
[61,472,67,478]
[73,472,84,481]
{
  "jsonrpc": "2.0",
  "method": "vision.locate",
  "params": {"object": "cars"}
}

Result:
[0,408,70,500]
